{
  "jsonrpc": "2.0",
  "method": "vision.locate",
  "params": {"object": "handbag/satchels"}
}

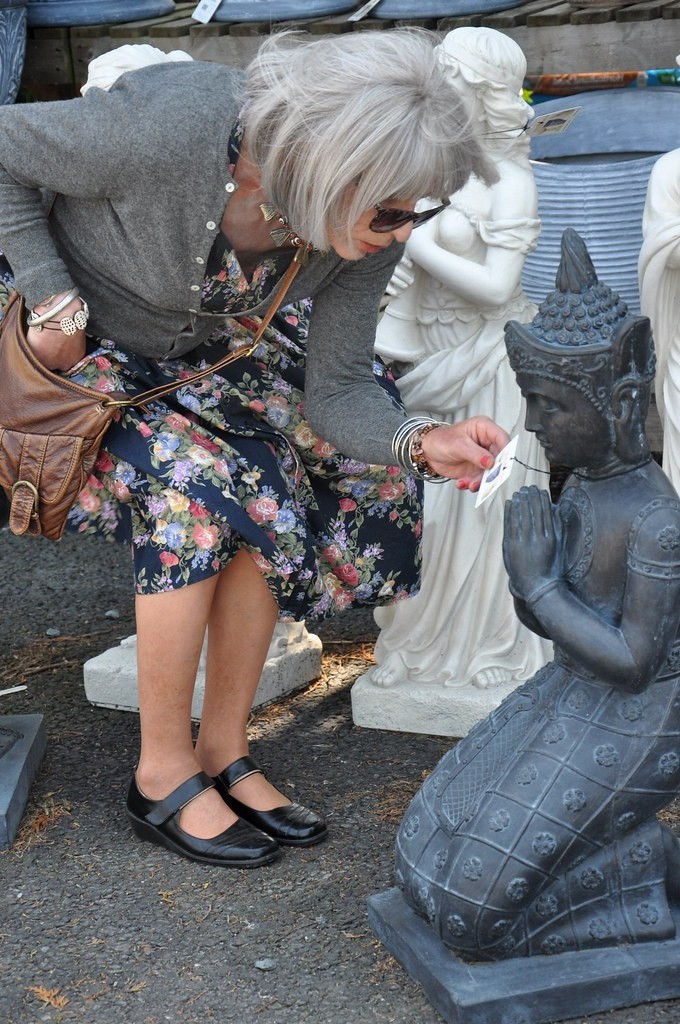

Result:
[0,289,137,543]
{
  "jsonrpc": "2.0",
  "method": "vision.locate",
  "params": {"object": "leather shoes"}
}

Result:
[125,769,279,868]
[215,754,329,846]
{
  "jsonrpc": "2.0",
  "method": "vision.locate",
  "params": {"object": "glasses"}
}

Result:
[352,176,452,233]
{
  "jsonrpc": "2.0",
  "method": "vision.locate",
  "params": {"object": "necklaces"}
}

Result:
[257,202,325,253]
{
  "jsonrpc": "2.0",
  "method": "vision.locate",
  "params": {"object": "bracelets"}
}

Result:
[391,417,453,484]
[27,289,89,337]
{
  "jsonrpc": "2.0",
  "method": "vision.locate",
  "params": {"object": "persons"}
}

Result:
[371,27,546,687]
[80,44,192,99]
[637,146,680,494]
[391,228,680,962]
[0,30,509,866]
